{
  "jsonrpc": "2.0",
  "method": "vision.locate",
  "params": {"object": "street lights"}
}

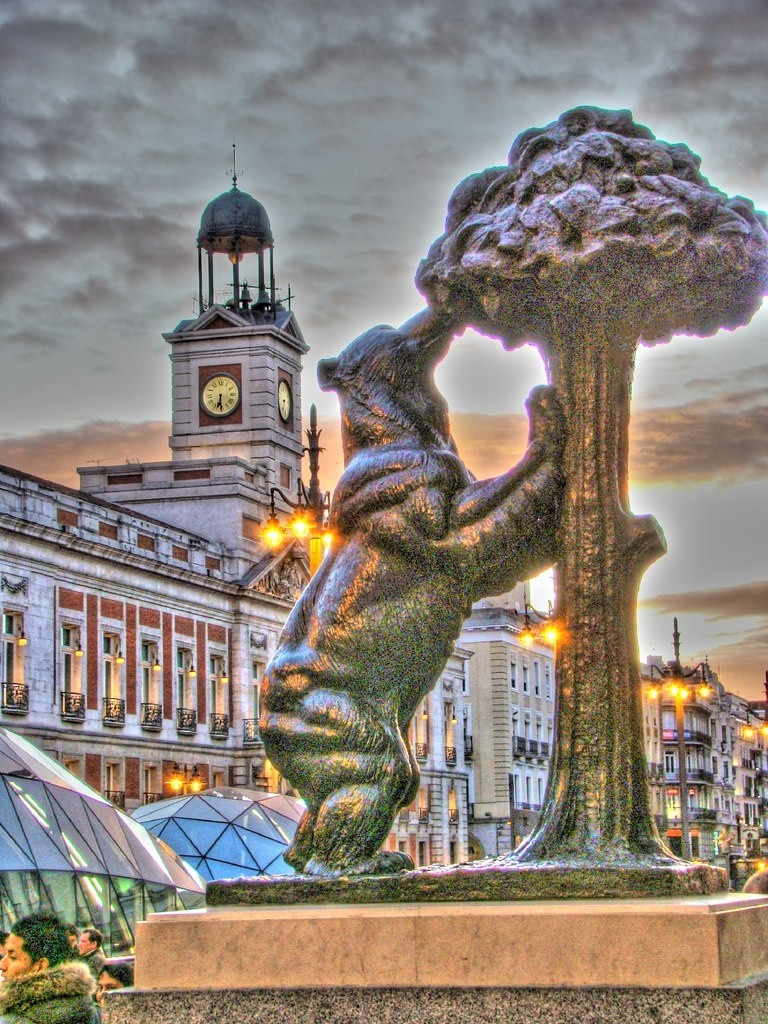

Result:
[646,662,715,862]
[261,477,330,576]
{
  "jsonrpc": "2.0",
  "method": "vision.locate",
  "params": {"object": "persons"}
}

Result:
[0,913,134,1024]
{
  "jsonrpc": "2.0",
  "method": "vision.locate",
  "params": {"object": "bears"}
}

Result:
[253,300,565,875]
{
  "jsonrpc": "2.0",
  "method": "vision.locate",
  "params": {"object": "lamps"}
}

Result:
[19,631,27,646]
[422,710,428,720]
[220,671,229,684]
[170,764,183,790]
[75,643,83,658]
[188,665,197,677]
[117,651,125,664]
[153,658,161,671]
[190,765,202,792]
[452,715,457,724]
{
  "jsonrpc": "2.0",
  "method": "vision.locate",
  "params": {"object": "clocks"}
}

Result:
[199,372,241,418]
[278,378,293,424]
